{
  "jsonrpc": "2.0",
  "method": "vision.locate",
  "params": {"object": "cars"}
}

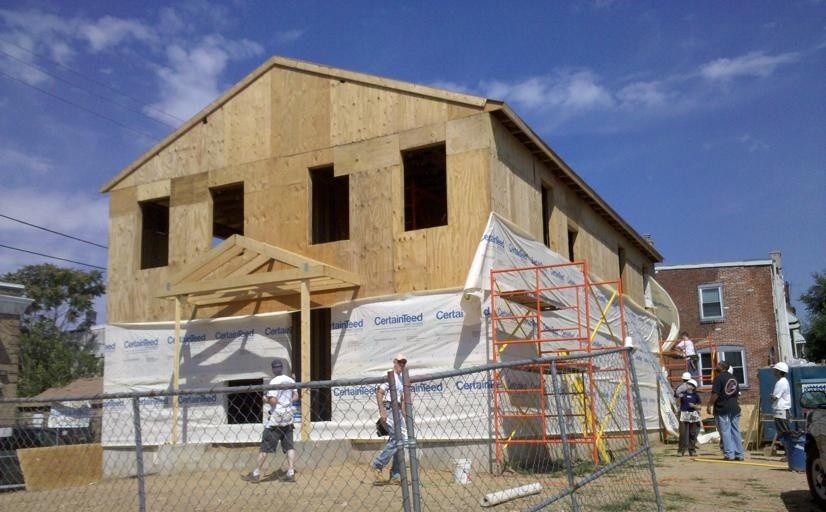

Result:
[800,391,826,506]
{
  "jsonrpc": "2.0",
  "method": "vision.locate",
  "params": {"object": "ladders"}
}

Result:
[557,348,616,466]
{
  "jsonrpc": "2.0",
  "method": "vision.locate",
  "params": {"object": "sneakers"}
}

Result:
[677,448,789,462]
[239,467,411,487]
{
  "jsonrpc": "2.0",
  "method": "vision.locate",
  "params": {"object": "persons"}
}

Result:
[707,359,744,462]
[674,379,702,456]
[768,361,794,466]
[240,358,299,483]
[673,331,698,370]
[368,352,413,488]
[674,372,692,399]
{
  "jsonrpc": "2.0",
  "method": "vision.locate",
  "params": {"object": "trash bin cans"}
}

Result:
[778,431,807,472]
[702,418,716,433]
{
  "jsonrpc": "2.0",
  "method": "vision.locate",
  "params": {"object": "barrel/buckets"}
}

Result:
[452,460,471,484]
[787,436,806,472]
[703,417,716,433]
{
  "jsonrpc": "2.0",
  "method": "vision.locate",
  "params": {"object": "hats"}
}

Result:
[271,359,283,367]
[393,354,408,363]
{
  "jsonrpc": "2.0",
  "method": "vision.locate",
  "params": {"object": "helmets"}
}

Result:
[681,371,692,381]
[772,361,789,373]
[727,365,734,375]
[688,379,698,387]
